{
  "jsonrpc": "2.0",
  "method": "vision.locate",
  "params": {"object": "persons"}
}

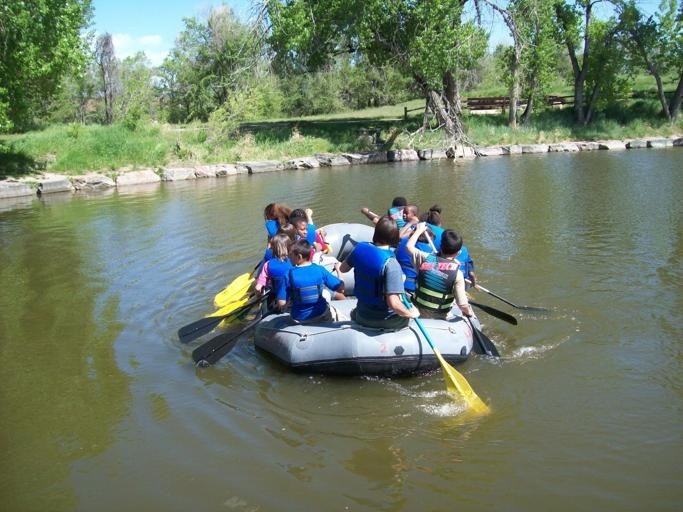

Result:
[340,216,421,329]
[253,202,346,326]
[360,195,482,321]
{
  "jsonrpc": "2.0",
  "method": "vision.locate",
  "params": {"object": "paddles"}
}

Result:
[465,277,549,313]
[402,294,491,417]
[468,301,516,326]
[178,273,278,367]
[422,232,498,358]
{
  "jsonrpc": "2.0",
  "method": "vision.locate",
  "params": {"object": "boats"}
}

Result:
[255,223,474,379]
